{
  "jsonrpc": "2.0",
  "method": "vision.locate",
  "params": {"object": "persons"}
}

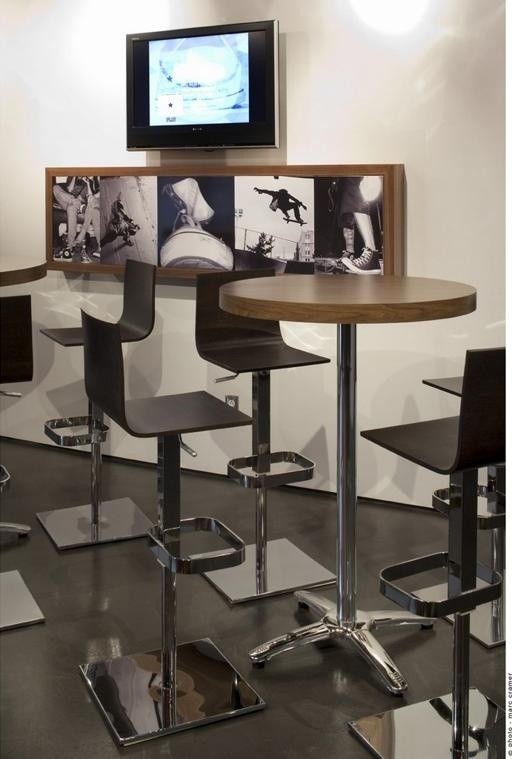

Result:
[52,175,101,264]
[333,177,382,275]
[254,187,307,222]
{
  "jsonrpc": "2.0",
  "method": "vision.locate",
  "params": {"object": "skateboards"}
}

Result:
[282,218,307,226]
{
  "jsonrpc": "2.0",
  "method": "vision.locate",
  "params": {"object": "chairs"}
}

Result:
[0,258,338,747]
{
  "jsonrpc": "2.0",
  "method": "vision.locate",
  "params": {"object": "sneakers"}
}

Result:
[79,204,86,213]
[330,247,382,275]
[53,246,101,264]
[160,178,234,272]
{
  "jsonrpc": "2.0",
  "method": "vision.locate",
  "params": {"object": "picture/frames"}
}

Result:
[43,163,410,284]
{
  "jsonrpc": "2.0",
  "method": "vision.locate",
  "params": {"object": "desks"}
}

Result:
[218,275,478,697]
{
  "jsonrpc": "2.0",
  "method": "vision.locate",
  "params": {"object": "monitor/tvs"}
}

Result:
[126,19,279,151]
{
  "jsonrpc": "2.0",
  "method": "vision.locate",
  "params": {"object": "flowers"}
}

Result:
[355,347,501,758]
